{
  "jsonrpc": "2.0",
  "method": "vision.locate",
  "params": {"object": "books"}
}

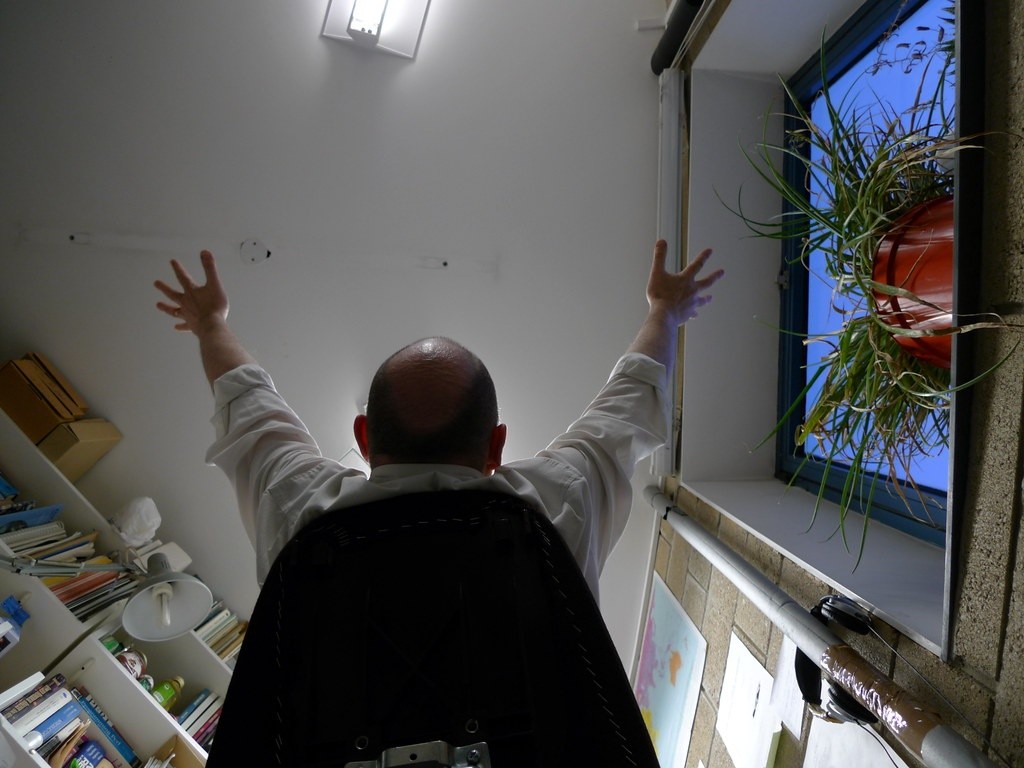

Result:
[0,480,247,768]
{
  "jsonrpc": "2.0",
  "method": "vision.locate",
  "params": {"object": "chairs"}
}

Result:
[205,490,660,768]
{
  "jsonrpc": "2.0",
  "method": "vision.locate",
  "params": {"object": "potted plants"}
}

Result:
[714,24,1024,574]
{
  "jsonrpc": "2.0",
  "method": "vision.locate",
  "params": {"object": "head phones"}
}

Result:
[794,594,882,726]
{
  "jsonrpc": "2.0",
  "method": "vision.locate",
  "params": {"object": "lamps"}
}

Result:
[0,552,213,642]
[319,0,431,61]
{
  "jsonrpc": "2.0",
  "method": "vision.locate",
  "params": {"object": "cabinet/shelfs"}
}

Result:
[0,408,178,678]
[0,601,249,768]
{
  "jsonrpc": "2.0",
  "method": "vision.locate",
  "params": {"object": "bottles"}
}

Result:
[151,675,185,712]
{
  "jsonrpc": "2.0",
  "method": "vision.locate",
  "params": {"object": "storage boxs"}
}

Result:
[0,351,91,447]
[37,416,123,485]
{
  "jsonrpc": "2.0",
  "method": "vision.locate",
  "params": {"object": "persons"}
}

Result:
[155,238,726,606]
